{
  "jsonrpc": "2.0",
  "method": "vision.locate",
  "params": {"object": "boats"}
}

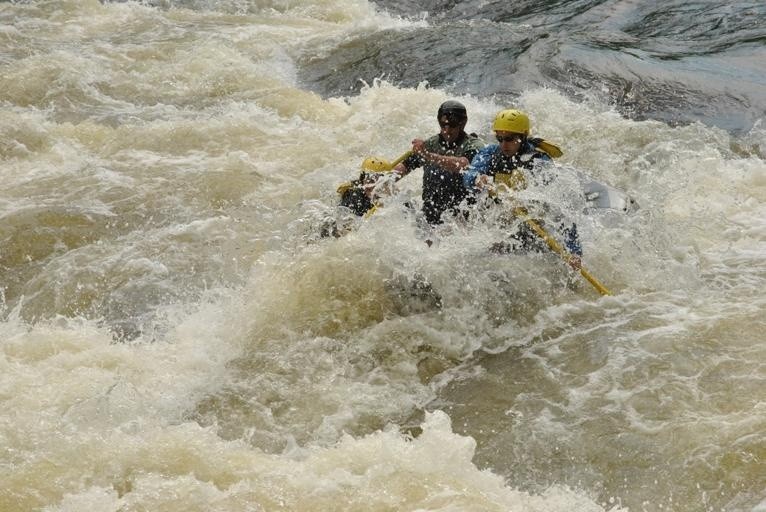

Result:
[383,166,640,298]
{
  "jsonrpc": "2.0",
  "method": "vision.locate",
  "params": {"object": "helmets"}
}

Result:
[435,100,468,118]
[360,155,394,175]
[491,109,531,138]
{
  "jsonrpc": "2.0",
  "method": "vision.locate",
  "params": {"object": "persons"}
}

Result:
[462,108,583,270]
[363,101,484,226]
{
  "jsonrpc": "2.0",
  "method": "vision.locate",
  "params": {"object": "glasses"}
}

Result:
[495,133,520,143]
[438,120,463,129]
